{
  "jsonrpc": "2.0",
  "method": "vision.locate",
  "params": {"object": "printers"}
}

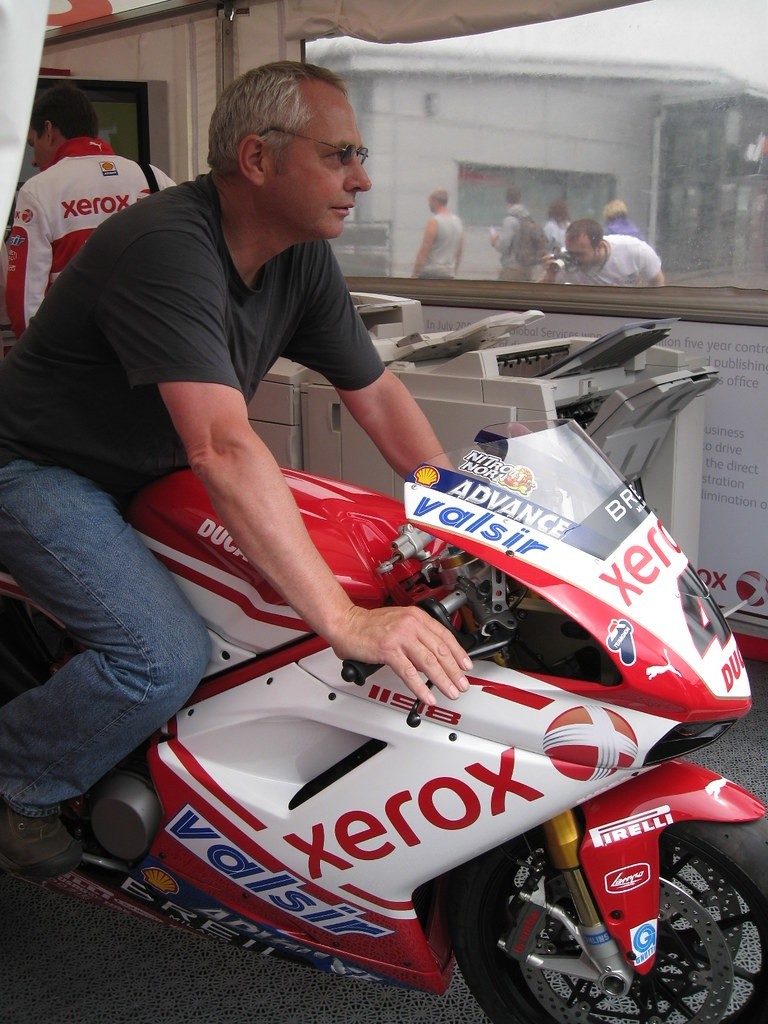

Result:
[243,289,722,574]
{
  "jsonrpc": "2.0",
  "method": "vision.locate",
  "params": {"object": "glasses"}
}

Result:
[259,126,369,166]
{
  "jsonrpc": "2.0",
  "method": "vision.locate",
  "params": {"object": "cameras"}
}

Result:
[548,252,575,273]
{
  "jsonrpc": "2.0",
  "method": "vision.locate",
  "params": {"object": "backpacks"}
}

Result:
[506,209,549,266]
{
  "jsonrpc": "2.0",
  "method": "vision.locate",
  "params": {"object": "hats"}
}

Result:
[603,197,629,222]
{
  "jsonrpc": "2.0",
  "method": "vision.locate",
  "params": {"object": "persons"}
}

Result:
[0,58,476,878]
[412,186,667,288]
[5,80,179,352]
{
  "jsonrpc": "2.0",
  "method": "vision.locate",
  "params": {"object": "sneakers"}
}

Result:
[0,798,82,879]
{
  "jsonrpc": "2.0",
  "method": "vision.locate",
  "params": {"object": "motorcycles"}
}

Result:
[1,418,768,1024]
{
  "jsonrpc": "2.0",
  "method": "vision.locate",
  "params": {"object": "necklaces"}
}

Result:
[580,242,609,278]
